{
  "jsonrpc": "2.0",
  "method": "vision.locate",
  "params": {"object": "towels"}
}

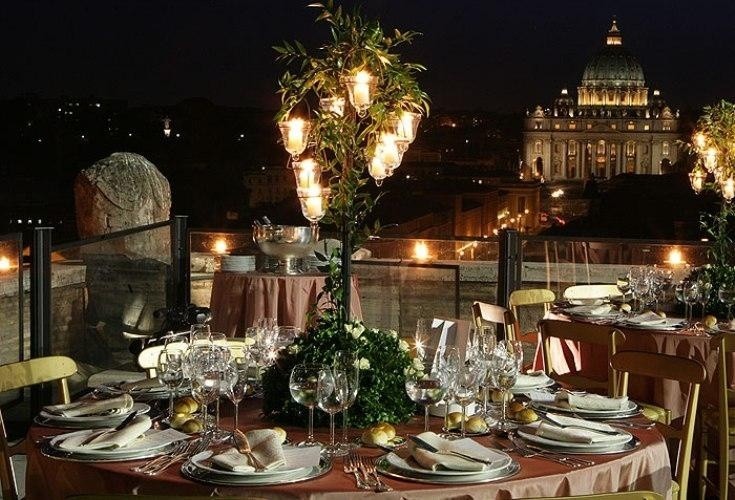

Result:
[564,303,612,315]
[625,310,666,326]
[555,390,623,410]
[408,430,489,471]
[536,412,629,444]
[109,375,165,390]
[44,392,134,416]
[515,368,551,386]
[58,415,152,449]
[211,428,285,471]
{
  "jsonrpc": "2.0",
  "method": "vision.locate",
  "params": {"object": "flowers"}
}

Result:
[682,265,735,320]
[259,323,424,437]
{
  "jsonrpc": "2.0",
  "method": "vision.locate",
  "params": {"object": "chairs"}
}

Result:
[562,285,624,304]
[610,350,706,500]
[534,320,627,399]
[510,289,555,374]
[468,301,517,346]
[717,332,735,500]
[0,354,78,500]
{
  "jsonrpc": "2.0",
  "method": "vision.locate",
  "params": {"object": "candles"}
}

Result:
[277,66,424,225]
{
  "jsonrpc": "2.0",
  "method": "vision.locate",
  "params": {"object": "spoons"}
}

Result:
[526,444,596,468]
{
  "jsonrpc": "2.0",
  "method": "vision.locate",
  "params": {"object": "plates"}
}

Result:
[518,421,634,450]
[387,449,513,478]
[517,426,642,455]
[32,414,150,427]
[302,257,342,274]
[180,458,334,488]
[373,455,523,484]
[532,400,645,419]
[540,400,637,414]
[191,447,305,477]
[41,436,180,462]
[39,402,150,421]
[50,431,173,455]
[509,378,550,385]
[102,380,197,399]
[220,254,255,273]
[512,379,556,391]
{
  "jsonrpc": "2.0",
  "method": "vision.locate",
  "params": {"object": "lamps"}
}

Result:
[677,100,734,265]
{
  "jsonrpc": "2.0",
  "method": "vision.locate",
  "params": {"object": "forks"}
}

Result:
[511,439,574,470]
[131,440,193,475]
[144,437,213,477]
[347,444,371,486]
[361,455,393,493]
[341,456,369,493]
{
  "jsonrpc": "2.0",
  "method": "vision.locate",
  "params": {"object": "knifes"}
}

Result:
[78,409,138,447]
[532,410,621,436]
[406,433,494,466]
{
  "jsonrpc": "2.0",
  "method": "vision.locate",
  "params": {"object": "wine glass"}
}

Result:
[194,363,222,448]
[718,285,734,325]
[653,270,676,314]
[192,331,228,345]
[640,265,658,310]
[490,348,520,431]
[673,284,686,305]
[156,349,185,425]
[470,345,496,416]
[164,336,189,400]
[625,265,641,310]
[334,349,361,450]
[499,337,524,403]
[189,325,212,347]
[226,358,250,433]
[403,362,444,434]
[290,363,335,446]
[242,317,299,365]
[315,369,347,458]
[635,277,649,312]
[631,274,651,316]
[206,350,239,429]
[183,343,215,412]
[697,283,711,321]
[618,273,631,309]
[682,282,699,322]
[449,369,483,440]
[472,325,496,349]
[431,344,460,435]
